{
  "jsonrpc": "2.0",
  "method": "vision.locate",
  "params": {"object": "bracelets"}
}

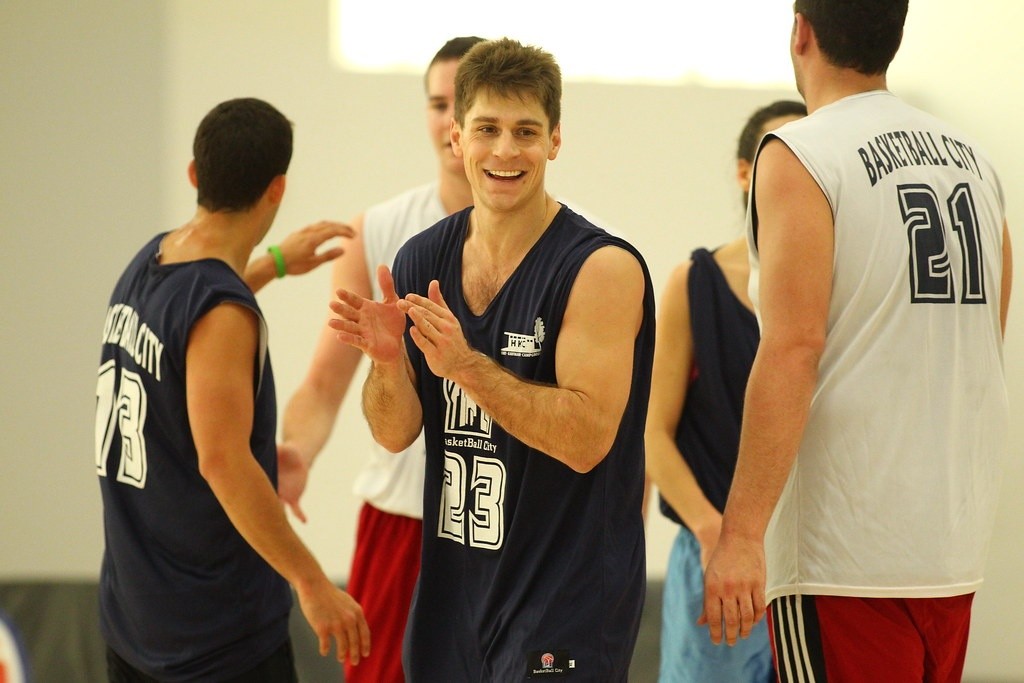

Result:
[267,244,286,278]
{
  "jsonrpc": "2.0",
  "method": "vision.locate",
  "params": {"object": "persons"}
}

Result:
[93,95,373,683]
[641,96,811,681]
[325,38,656,681]
[274,35,492,682]
[692,0,1016,683]
